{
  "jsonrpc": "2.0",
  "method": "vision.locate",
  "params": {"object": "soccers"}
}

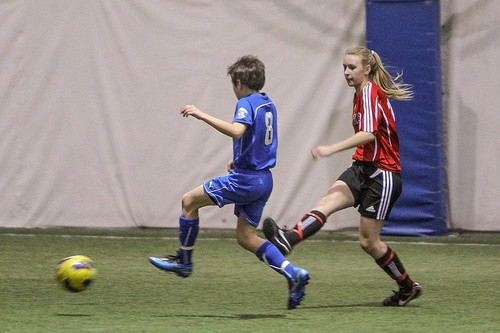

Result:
[54,255,98,292]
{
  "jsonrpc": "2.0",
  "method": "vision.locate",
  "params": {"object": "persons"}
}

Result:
[148,52,310,309]
[263,47,423,307]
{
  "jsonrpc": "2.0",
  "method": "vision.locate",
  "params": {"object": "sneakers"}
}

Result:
[149,255,192,278]
[382,281,421,307]
[263,218,293,257]
[287,267,310,310]
